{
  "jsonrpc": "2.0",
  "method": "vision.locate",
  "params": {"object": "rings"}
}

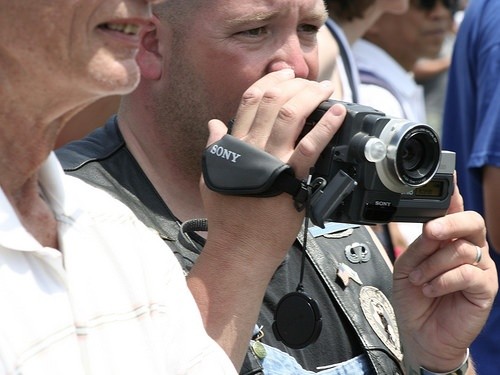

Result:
[471,245,482,266]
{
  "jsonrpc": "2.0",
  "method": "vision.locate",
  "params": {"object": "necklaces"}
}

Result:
[323,20,358,106]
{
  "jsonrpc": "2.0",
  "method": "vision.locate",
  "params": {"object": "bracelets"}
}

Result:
[400,348,469,375]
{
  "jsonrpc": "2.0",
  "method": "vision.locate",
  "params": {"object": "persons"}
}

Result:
[353,0,459,263]
[441,0,500,375]
[321,0,409,103]
[0,0,240,375]
[55,0,498,375]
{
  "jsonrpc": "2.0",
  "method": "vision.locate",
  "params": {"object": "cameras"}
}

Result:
[227,99,455,225]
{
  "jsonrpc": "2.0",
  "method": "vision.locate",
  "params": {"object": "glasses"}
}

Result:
[414,0,459,14]
